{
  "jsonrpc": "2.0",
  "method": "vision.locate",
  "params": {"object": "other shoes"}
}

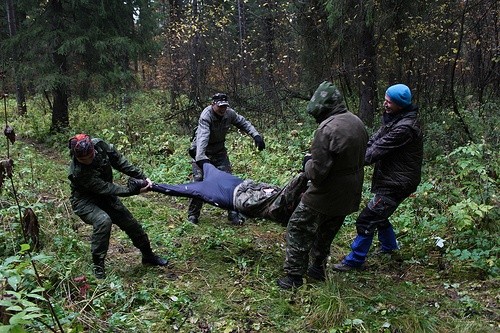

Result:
[188,215,198,224]
[142,255,168,266]
[278,274,304,290]
[94,266,106,279]
[230,216,243,226]
[332,261,364,273]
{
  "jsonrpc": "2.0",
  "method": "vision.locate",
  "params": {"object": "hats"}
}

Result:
[212,92,230,107]
[68,133,95,158]
[385,83,413,108]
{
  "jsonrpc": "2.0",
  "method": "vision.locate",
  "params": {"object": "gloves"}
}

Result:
[254,135,265,151]
[196,159,211,169]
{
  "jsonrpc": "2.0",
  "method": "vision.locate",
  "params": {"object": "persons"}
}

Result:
[332,84,423,272]
[128,148,311,227]
[277,80,369,288]
[67,134,168,279]
[187,93,266,225]
